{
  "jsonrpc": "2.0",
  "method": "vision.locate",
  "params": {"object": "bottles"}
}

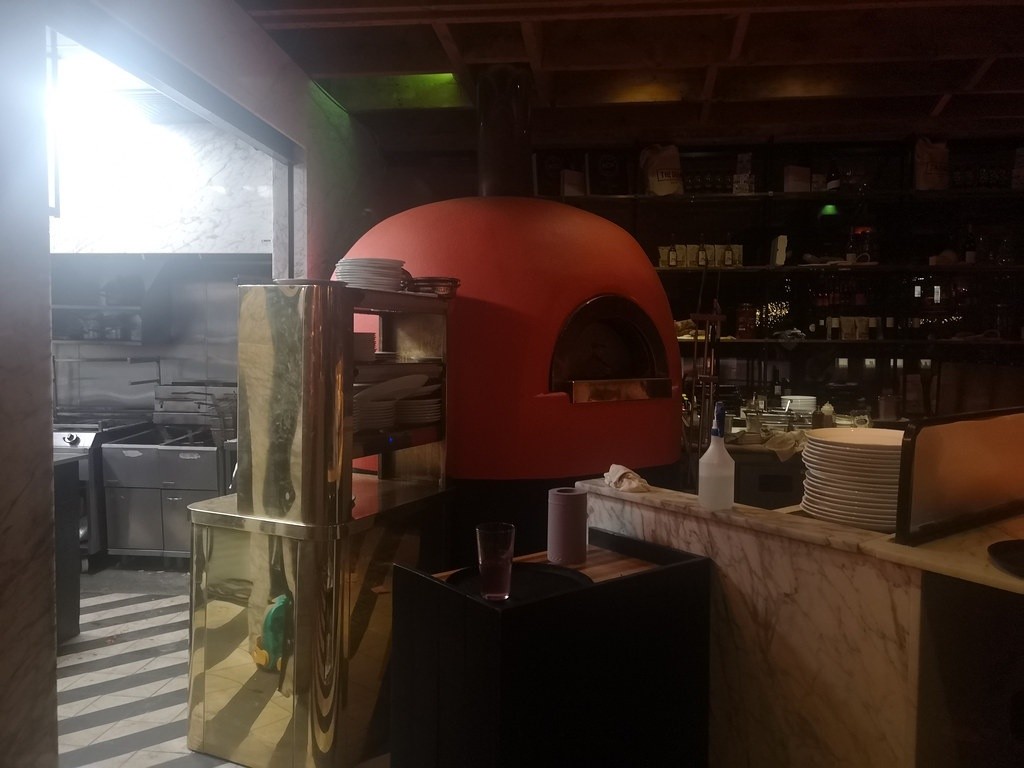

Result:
[127,311,144,343]
[697,233,707,266]
[724,232,733,266]
[747,391,761,414]
[667,232,678,266]
[858,229,872,260]
[827,155,840,190]
[845,223,858,263]
[813,401,834,426]
[770,368,782,408]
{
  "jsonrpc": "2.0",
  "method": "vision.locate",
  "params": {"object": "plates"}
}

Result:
[273,279,347,287]
[354,331,399,364]
[334,258,405,292]
[353,374,442,433]
[801,427,905,532]
[780,395,817,412]
[409,356,444,363]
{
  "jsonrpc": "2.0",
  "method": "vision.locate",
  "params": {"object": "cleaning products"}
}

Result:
[697,401,735,512]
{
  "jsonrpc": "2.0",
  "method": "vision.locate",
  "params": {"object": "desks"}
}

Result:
[391,525,710,768]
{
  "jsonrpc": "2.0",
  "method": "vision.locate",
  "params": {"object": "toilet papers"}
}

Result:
[546,487,588,566]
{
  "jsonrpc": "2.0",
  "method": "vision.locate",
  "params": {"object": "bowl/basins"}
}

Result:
[103,326,125,341]
[410,277,461,298]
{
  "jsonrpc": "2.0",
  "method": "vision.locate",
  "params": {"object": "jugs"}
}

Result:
[77,317,101,340]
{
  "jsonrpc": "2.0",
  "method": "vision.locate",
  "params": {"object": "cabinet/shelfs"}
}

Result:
[50,304,142,347]
[523,135,1024,426]
[102,447,225,558]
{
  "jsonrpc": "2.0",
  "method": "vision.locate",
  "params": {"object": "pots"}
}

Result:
[742,407,794,434]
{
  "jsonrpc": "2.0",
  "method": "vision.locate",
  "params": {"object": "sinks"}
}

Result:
[101,426,199,449]
[162,425,237,451]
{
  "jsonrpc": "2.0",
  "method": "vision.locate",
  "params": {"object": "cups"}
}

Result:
[725,414,736,433]
[475,522,516,601]
